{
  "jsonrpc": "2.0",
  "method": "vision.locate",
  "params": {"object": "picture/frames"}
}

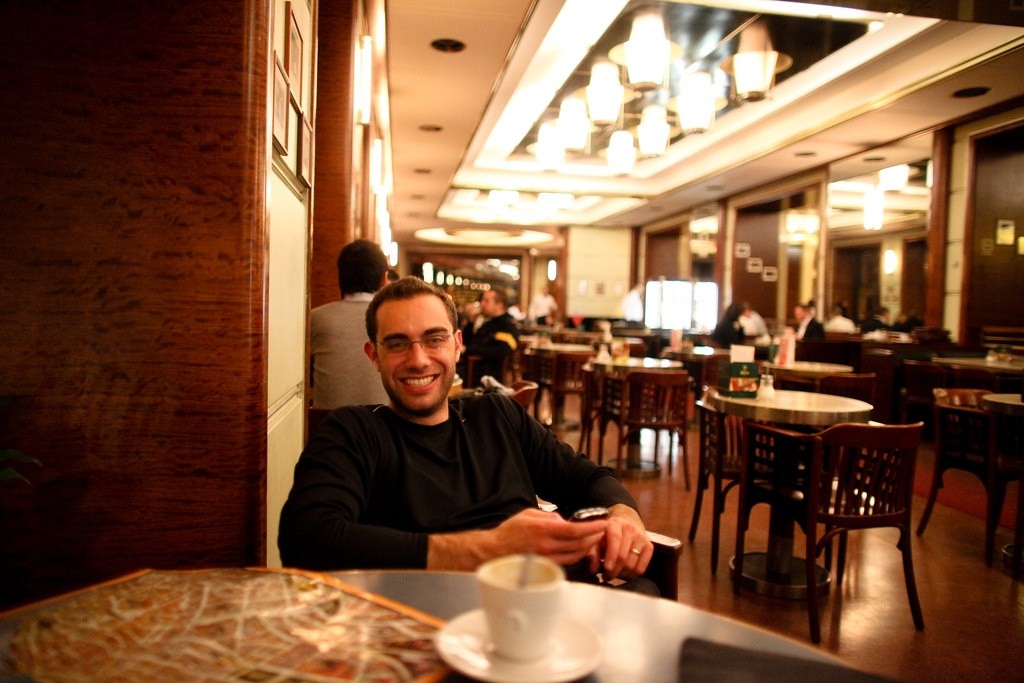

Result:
[273,0,316,202]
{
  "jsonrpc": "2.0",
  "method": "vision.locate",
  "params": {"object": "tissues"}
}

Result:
[715,343,760,399]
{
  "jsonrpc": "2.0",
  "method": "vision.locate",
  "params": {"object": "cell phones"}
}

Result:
[569,507,608,523]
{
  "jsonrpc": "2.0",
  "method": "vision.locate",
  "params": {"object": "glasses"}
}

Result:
[376,334,455,352]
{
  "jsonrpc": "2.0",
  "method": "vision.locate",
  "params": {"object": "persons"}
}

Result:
[384,263,561,388]
[310,238,397,412]
[276,274,663,601]
[707,295,927,350]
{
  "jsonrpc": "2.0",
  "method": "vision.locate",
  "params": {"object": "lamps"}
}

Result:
[526,4,794,175]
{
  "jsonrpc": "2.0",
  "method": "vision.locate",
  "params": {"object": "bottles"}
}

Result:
[780,328,795,366]
[597,345,611,362]
[757,374,776,400]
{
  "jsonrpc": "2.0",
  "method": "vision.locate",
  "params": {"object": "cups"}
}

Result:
[477,556,564,660]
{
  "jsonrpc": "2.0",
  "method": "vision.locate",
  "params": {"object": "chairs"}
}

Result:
[467,325,1024,644]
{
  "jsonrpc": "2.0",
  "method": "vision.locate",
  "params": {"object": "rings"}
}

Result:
[630,547,642,556]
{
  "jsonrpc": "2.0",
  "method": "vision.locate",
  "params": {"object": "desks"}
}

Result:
[519,342,594,431]
[581,355,686,482]
[702,388,875,602]
[315,571,868,683]
[554,332,649,357]
[757,360,853,388]
[929,356,1024,394]
[663,345,730,433]
[980,393,1024,581]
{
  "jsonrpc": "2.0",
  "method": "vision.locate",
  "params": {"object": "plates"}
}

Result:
[434,608,604,683]
